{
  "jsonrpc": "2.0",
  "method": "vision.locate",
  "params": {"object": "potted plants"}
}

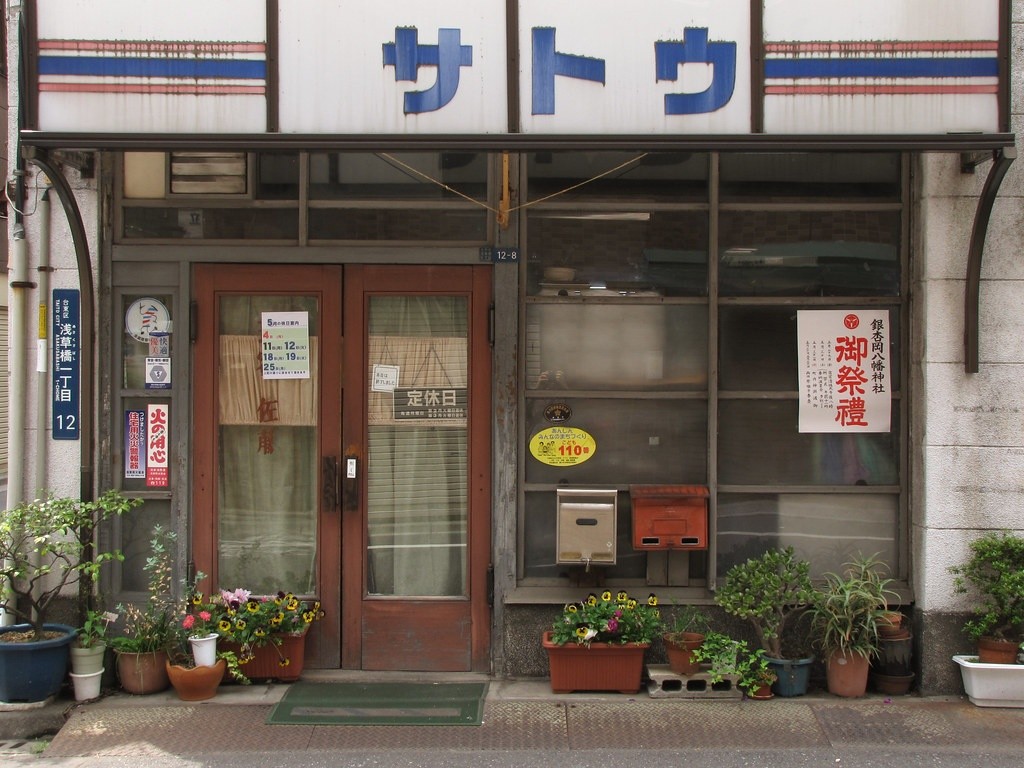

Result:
[70,610,108,675]
[942,528,1024,664]
[688,543,903,700]
[665,594,715,674]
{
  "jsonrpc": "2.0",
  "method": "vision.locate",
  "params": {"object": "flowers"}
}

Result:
[550,592,665,650]
[0,490,322,698]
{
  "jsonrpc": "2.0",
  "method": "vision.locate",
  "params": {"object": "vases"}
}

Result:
[187,633,220,666]
[873,632,916,674]
[69,667,106,701]
[874,671,915,696]
[0,624,76,702]
[164,652,225,702]
[543,630,647,694]
[952,654,1024,708]
[112,645,176,696]
[879,610,901,635]
[218,621,311,684]
